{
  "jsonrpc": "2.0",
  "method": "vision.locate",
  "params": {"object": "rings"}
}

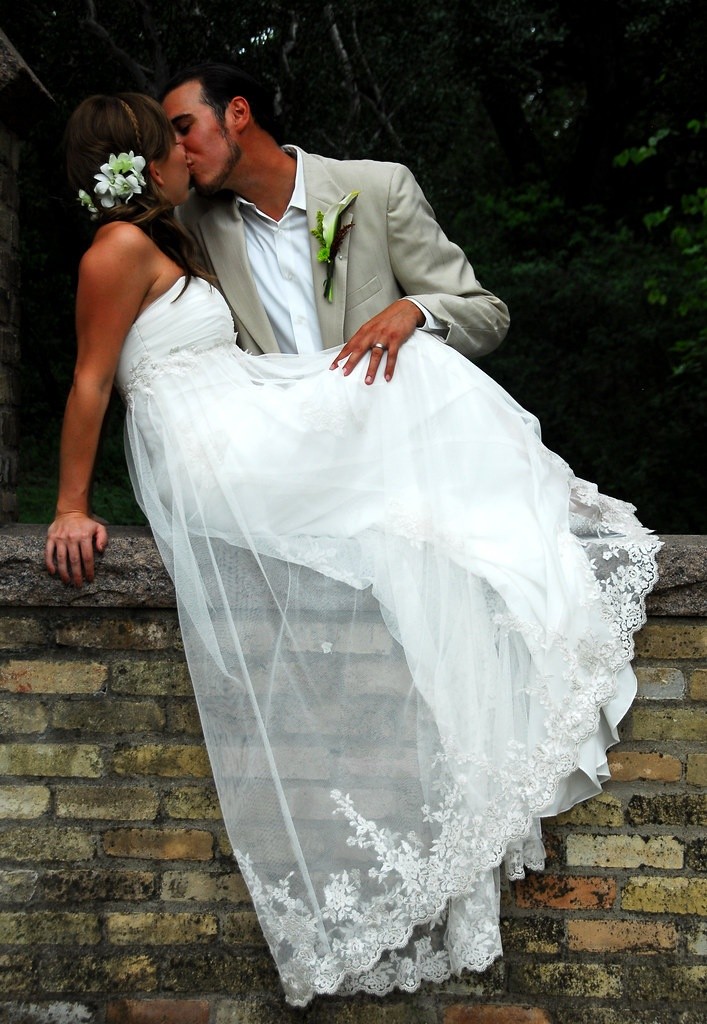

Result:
[372,342,385,351]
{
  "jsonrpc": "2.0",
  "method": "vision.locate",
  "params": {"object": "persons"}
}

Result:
[158,61,514,976]
[47,94,665,1011]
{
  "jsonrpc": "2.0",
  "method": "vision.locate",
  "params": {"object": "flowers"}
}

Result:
[94,149,148,203]
[315,189,363,305]
[78,190,97,218]
